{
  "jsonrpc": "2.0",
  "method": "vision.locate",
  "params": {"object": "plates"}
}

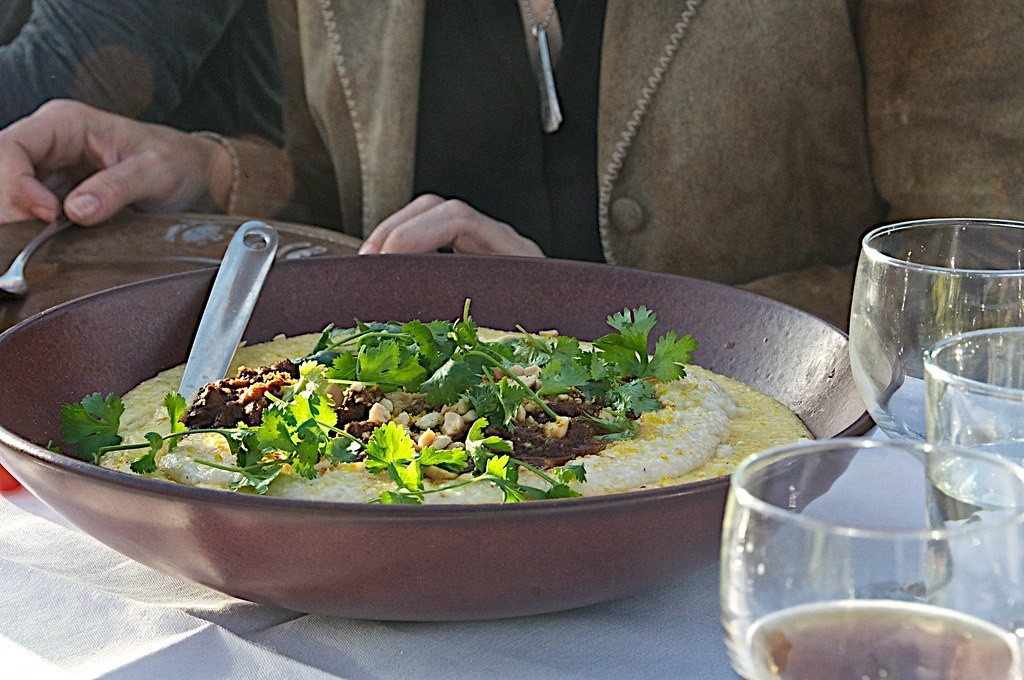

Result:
[0,255,902,621]
[0,212,366,336]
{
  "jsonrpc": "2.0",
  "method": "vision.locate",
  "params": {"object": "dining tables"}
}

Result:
[0,370,1023,680]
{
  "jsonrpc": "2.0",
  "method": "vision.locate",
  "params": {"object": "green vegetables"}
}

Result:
[42,298,697,503]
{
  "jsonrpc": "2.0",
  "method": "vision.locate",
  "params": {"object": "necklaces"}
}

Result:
[524,0,563,135]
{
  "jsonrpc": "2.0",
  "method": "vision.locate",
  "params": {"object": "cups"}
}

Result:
[848,218,1024,464]
[922,328,1024,633]
[718,437,1024,680]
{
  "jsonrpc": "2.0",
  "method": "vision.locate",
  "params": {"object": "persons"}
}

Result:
[0,0,1024,385]
[0,1,277,132]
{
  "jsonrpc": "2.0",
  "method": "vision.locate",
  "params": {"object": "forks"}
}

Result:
[0,218,74,295]
